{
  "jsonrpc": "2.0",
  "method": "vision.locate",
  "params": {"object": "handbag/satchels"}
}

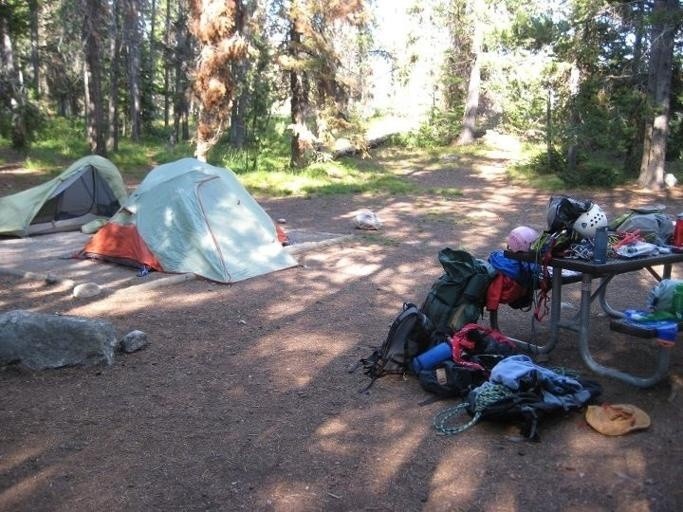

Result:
[489,247,547,289]
[488,273,548,313]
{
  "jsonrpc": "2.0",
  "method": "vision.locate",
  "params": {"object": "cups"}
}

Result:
[592,225,609,264]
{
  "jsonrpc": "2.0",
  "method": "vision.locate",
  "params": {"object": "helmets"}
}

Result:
[507,225,540,254]
[573,201,608,238]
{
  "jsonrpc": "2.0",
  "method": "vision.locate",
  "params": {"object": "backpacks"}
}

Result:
[419,359,486,399]
[377,302,435,375]
[608,206,676,248]
[423,246,487,332]
[464,354,600,431]
[448,322,528,371]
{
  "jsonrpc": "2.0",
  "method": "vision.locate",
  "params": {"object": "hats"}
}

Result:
[584,401,652,436]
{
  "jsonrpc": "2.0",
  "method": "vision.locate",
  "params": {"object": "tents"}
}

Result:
[70,157,302,281]
[0,153,126,238]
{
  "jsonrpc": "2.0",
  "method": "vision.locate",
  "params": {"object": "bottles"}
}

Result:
[673,212,683,248]
[411,342,452,375]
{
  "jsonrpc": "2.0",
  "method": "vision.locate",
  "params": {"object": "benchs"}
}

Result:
[609,312,682,389]
[490,266,581,354]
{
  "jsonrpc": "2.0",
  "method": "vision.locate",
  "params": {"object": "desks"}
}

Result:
[488,228,682,389]
